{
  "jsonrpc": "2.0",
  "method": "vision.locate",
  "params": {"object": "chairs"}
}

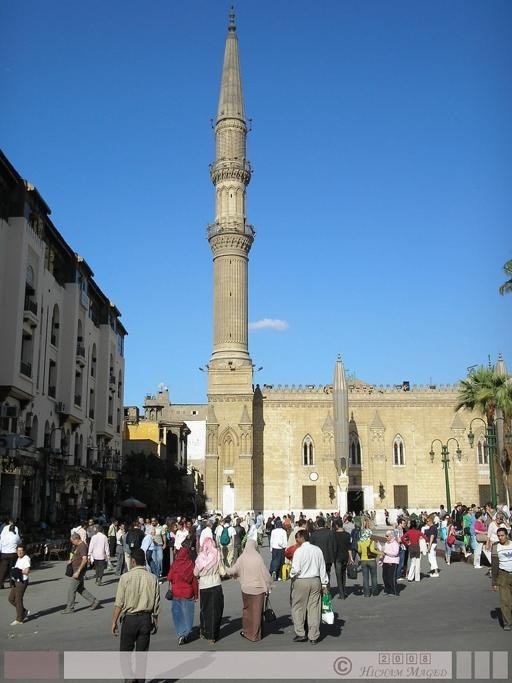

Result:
[29,542,71,561]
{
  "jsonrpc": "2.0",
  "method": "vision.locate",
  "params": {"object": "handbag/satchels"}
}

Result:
[66,563,73,576]
[476,534,488,541]
[448,534,455,544]
[367,541,377,558]
[265,598,276,622]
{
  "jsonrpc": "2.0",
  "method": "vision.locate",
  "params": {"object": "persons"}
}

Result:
[1,501,512,643]
[113,547,161,683]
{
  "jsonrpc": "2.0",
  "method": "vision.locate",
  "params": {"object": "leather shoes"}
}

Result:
[293,636,317,644]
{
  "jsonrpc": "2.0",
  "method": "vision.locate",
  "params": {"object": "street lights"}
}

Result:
[430,436,462,514]
[468,417,511,508]
[99,455,113,510]
[46,427,69,524]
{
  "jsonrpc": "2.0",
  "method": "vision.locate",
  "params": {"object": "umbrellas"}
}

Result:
[116,495,148,521]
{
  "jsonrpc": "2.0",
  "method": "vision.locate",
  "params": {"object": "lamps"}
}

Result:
[224,469,235,483]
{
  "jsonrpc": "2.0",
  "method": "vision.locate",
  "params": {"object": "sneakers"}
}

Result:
[10,611,31,626]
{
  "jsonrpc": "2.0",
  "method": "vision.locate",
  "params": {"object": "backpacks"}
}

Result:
[220,525,231,545]
